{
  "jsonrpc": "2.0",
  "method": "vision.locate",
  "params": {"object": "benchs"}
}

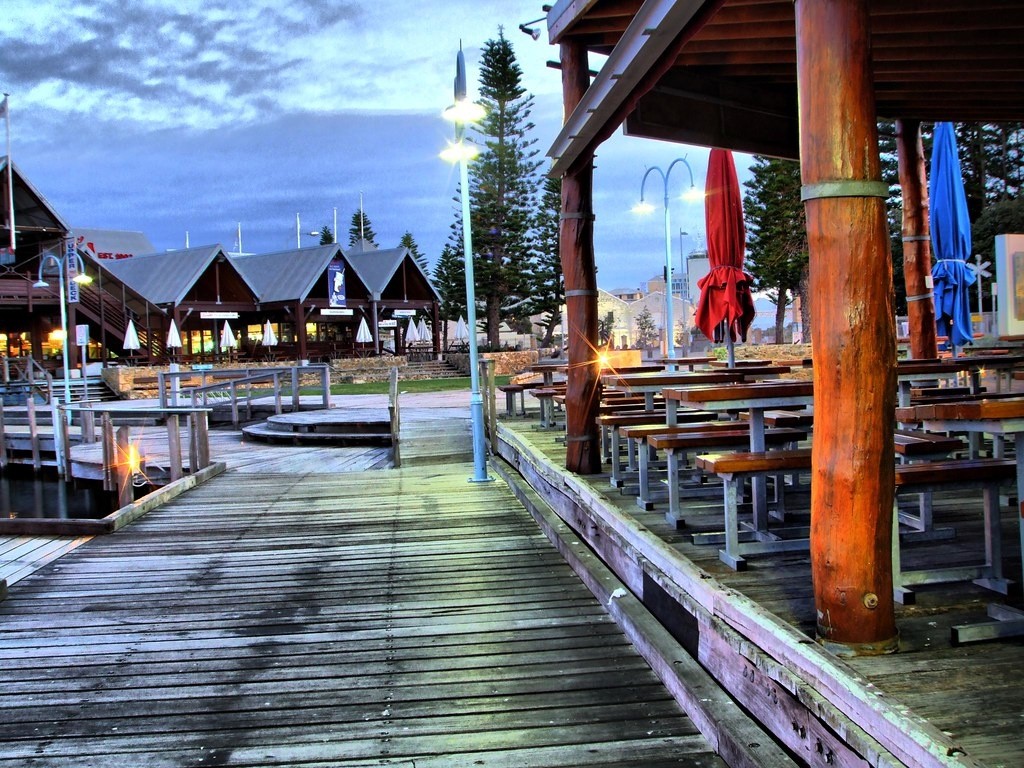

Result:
[501,370,1023,604]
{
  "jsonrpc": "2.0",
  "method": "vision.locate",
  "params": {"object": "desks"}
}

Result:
[524,334,1024,544]
[894,396,1024,644]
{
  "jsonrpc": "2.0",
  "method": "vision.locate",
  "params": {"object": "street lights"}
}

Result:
[632,152,700,373]
[679,226,689,334]
[436,39,495,483]
[296,212,321,250]
[31,250,95,427]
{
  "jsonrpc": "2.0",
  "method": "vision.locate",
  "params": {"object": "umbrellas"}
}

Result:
[405,317,433,345]
[929,123,977,358]
[453,315,470,345]
[167,319,183,355]
[696,149,756,369]
[355,317,373,348]
[220,320,238,362]
[262,319,278,353]
[122,319,141,357]
